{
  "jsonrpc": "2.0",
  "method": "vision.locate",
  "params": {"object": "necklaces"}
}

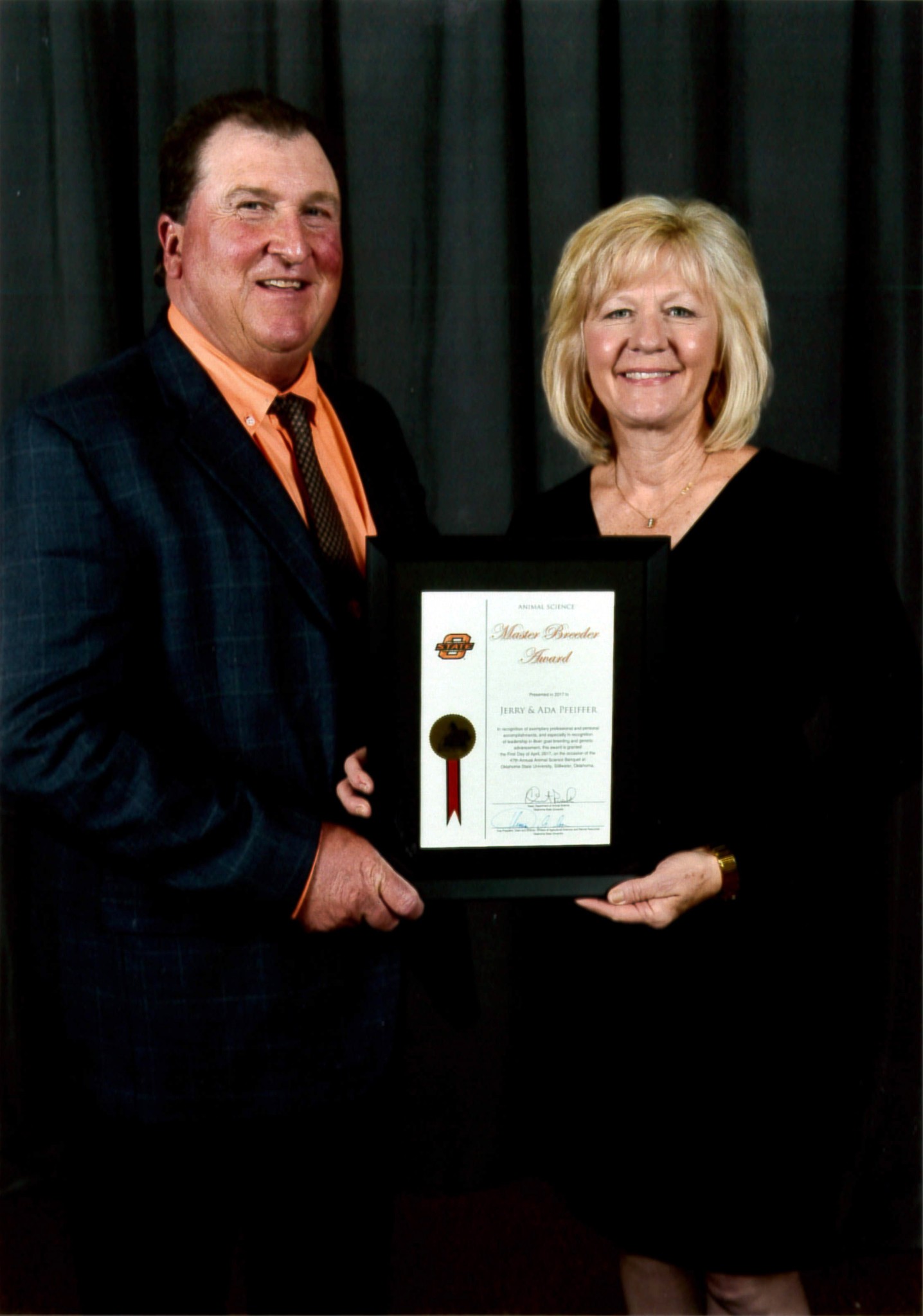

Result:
[615,452,709,527]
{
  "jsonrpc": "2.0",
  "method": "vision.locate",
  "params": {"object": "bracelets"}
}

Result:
[696,846,739,900]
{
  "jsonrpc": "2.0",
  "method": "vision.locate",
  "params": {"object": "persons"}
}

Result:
[334,189,893,1316]
[0,87,436,1316]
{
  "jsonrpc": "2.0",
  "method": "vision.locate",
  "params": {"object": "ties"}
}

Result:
[266,393,368,623]
